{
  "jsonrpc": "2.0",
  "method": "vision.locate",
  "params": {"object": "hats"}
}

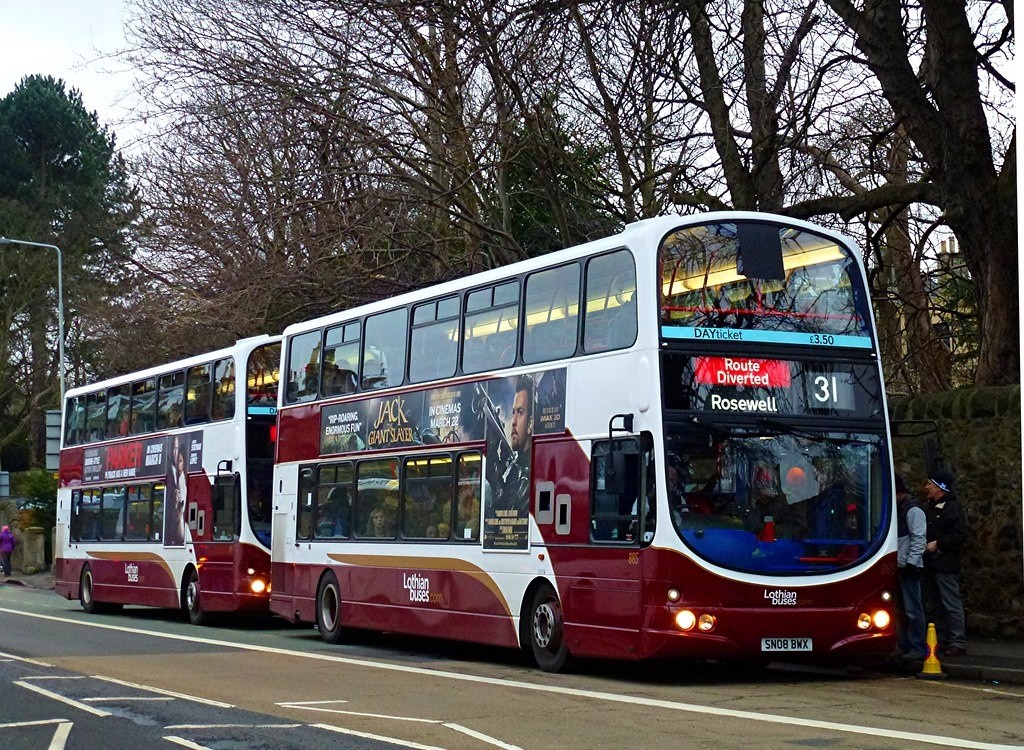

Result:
[386,481,398,494]
[930,471,955,493]
[3,525,9,531]
[845,503,856,513]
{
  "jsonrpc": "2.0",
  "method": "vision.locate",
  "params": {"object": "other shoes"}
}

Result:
[903,650,925,661]
[939,644,965,660]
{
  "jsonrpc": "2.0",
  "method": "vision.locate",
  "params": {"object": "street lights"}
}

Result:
[0,235,65,413]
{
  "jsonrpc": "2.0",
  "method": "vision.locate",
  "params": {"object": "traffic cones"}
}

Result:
[915,621,947,681]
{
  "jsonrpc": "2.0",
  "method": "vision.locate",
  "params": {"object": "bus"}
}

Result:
[269,211,946,676]
[52,333,284,627]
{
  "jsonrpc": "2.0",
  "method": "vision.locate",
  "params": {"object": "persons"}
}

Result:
[111,379,226,440]
[166,435,187,545]
[316,465,479,540]
[751,472,808,538]
[926,473,970,656]
[632,466,686,539]
[845,503,860,539]
[606,291,636,348]
[0,526,15,576]
[301,315,578,396]
[894,474,930,660]
[487,374,532,549]
